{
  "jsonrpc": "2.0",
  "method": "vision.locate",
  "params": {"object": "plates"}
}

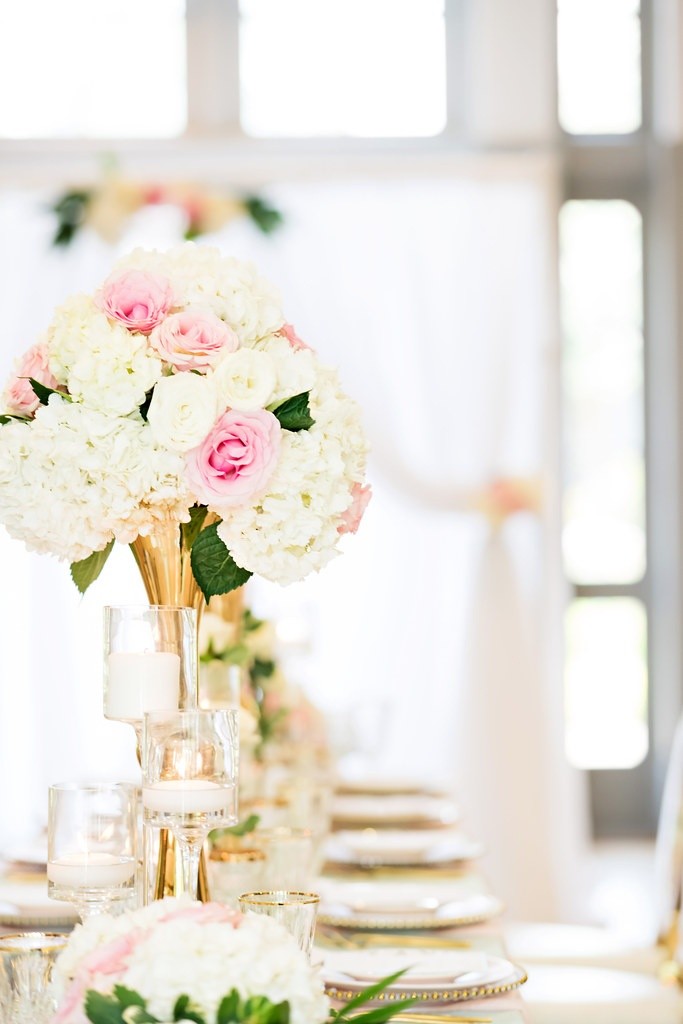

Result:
[321,780,528,1005]
[3,895,80,926]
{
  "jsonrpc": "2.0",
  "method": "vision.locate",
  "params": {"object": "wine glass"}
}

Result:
[43,601,242,902]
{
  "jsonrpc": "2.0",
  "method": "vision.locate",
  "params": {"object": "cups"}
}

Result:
[3,886,320,1024]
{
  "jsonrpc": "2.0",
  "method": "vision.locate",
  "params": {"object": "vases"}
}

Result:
[102,601,243,914]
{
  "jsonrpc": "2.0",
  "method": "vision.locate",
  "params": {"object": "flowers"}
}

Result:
[47,889,417,1024]
[0,139,374,903]
